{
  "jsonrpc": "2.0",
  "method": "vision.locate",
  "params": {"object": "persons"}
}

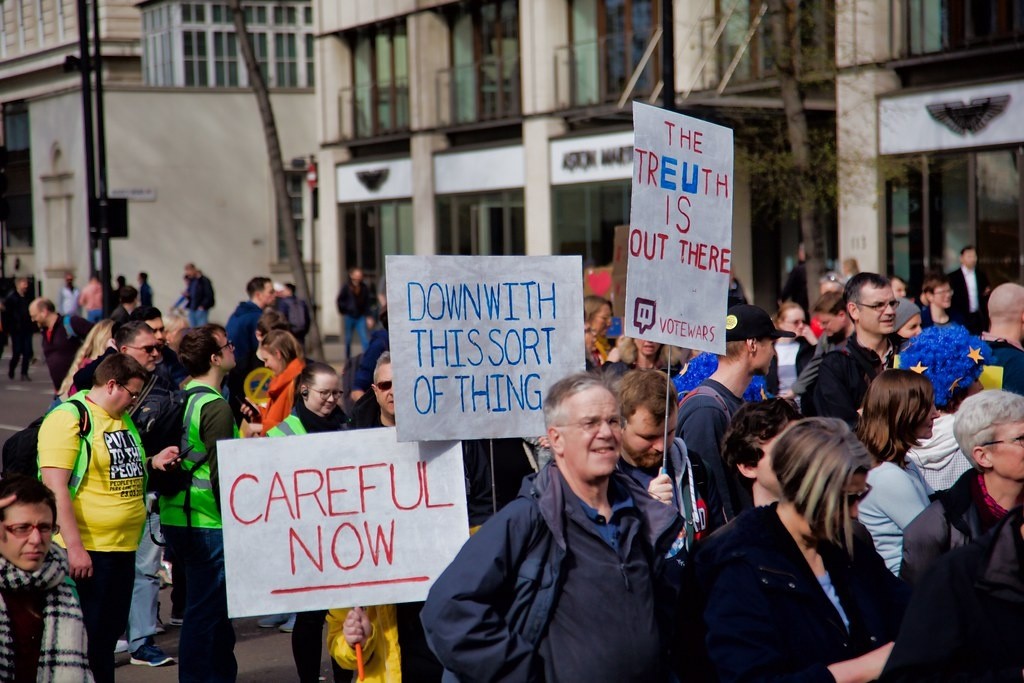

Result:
[0,245,1024,683]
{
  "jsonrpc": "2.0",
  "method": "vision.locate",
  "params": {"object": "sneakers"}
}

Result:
[256,611,289,627]
[129,645,174,666]
[278,613,296,632]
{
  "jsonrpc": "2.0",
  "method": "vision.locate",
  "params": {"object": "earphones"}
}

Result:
[302,390,308,396]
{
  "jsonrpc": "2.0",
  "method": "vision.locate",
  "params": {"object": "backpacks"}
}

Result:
[3,395,92,498]
[130,384,233,499]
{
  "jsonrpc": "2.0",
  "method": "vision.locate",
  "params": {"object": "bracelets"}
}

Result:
[147,458,154,470]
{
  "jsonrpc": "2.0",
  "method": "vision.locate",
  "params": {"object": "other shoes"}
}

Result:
[8,361,32,382]
[170,617,184,626]
[114,639,129,654]
[155,615,167,633]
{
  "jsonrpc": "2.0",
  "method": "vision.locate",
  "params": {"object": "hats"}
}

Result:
[726,305,797,342]
[895,295,921,333]
[899,324,992,409]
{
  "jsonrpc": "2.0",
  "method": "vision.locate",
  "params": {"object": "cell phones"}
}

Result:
[166,444,195,465]
[237,395,259,415]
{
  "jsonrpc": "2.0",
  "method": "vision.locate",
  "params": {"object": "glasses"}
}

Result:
[846,480,873,506]
[116,381,142,402]
[583,312,613,336]
[3,522,61,537]
[219,339,234,351]
[980,434,1024,450]
[789,274,954,328]
[127,344,163,354]
[305,385,344,402]
[552,415,629,436]
[375,380,392,393]
